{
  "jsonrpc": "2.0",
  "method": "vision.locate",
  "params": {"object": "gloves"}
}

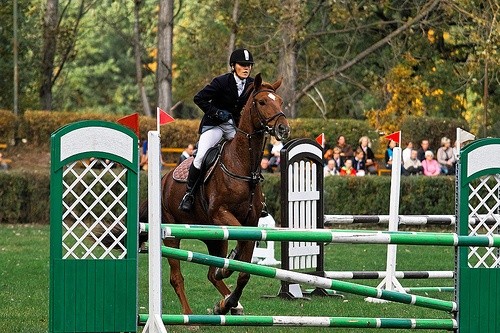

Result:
[216,109,229,123]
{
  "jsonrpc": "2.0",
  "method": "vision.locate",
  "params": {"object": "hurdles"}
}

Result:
[50,119,500,333]
[259,137,500,301]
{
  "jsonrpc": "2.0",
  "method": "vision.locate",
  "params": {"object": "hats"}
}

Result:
[441,137,451,145]
[425,151,434,159]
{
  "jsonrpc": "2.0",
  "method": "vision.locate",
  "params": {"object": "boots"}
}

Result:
[181,160,203,210]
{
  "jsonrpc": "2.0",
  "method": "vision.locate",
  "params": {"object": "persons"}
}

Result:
[438,137,457,175]
[403,149,422,176]
[417,140,433,162]
[402,140,414,162]
[422,151,441,176]
[334,137,353,167]
[178,49,255,212]
[358,136,378,173]
[178,136,458,177]
[384,139,396,169]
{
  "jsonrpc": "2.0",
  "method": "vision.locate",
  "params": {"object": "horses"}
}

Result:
[87,74,291,315]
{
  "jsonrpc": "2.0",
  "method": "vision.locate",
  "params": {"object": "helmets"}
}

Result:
[230,49,255,66]
